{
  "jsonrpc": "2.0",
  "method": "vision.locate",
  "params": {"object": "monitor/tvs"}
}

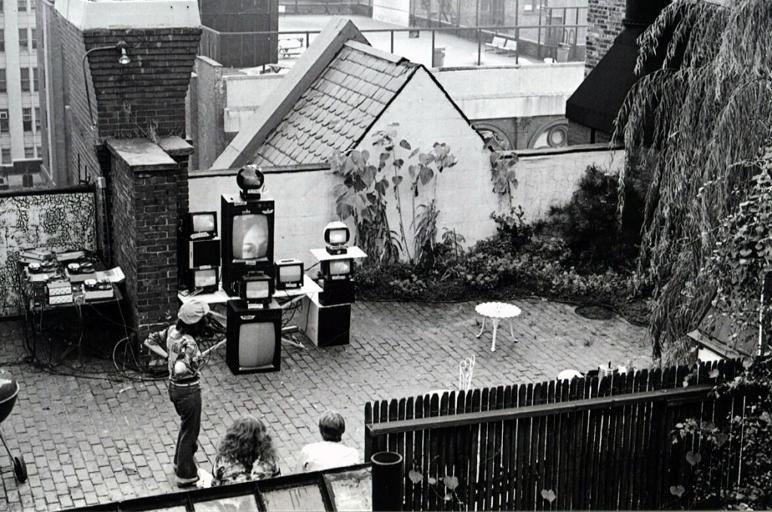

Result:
[189,193,355,375]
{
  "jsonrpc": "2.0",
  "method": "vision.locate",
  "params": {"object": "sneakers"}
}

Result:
[173,463,200,488]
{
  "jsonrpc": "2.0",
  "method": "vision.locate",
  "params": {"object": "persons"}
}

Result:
[233,215,268,259]
[143,297,211,484]
[295,409,360,475]
[210,413,281,486]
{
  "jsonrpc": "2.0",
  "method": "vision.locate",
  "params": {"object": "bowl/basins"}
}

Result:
[84,279,98,290]
[27,263,40,273]
[68,263,80,274]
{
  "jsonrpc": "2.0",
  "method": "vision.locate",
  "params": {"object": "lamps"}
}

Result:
[118,46,131,65]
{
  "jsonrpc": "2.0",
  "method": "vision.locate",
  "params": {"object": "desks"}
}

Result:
[474,301,520,351]
[177,274,325,359]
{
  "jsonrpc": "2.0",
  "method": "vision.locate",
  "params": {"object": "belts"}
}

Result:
[171,379,200,387]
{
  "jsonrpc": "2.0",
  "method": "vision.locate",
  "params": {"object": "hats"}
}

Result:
[177,299,209,324]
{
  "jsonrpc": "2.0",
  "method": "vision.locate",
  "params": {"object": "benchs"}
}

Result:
[485,36,516,57]
[690,307,759,368]
